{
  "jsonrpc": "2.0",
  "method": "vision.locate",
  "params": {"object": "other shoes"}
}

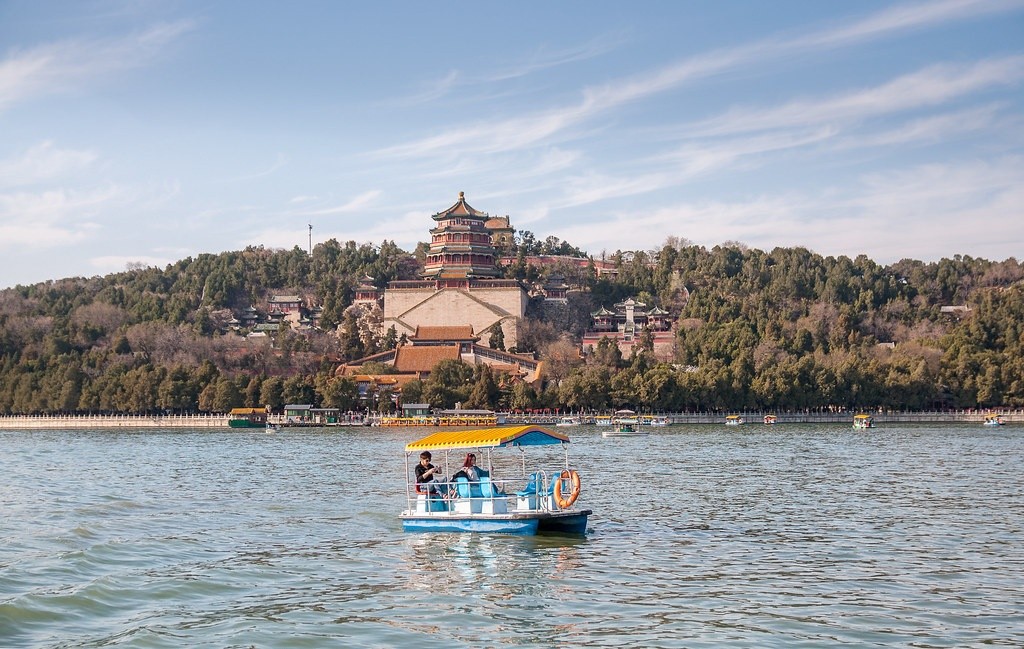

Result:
[442,494,452,504]
[449,489,459,499]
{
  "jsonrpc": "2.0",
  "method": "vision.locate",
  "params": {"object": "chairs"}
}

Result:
[516,473,542,510]
[538,473,565,511]
[455,476,484,514]
[414,476,444,511]
[479,476,508,514]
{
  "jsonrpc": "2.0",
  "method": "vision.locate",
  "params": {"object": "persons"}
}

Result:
[450,453,505,495]
[413,450,458,504]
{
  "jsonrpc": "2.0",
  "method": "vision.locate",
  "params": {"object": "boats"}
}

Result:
[249,408,272,427]
[398,425,593,536]
[764,415,777,425]
[651,416,671,426]
[983,414,1003,427]
[602,421,650,437]
[556,416,656,428]
[853,415,876,430]
[228,409,255,428]
[725,415,741,426]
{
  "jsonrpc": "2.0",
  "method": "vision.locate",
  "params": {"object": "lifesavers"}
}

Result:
[552,470,581,506]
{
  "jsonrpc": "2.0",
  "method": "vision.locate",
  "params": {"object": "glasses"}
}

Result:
[423,459,431,462]
